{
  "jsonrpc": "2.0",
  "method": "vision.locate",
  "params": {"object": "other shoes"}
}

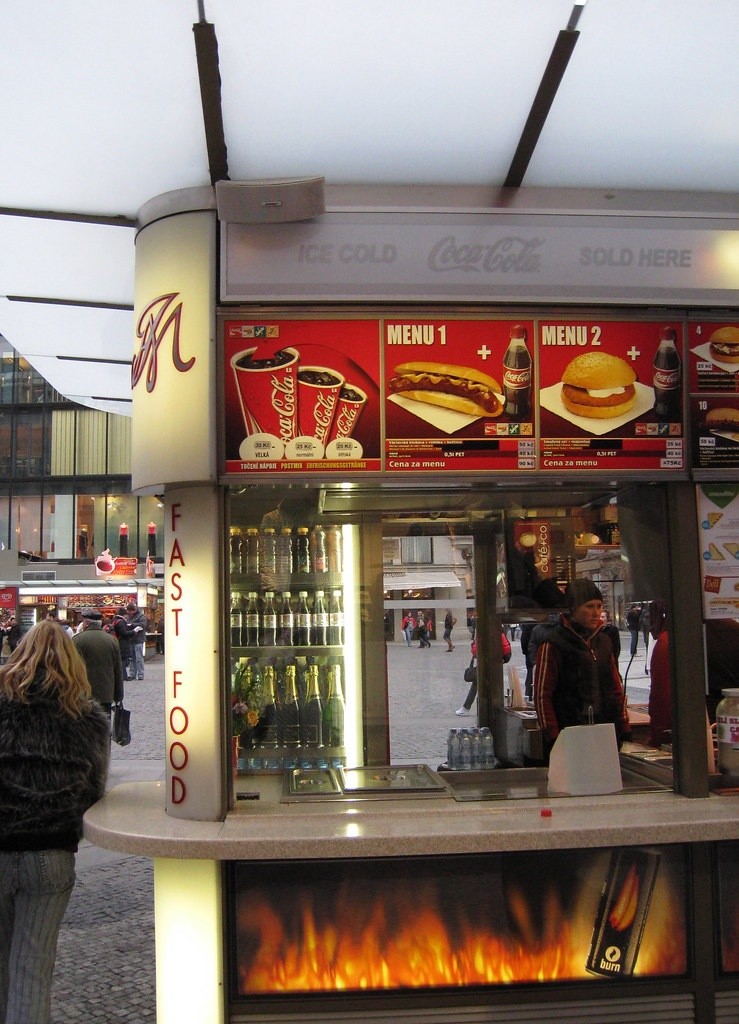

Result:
[125,679,132,681]
[634,653,642,657]
[139,677,143,680]
[128,676,135,679]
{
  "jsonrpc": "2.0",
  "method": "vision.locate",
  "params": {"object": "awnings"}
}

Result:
[383,571,462,591]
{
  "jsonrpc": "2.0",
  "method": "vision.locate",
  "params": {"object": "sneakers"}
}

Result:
[456,708,469,716]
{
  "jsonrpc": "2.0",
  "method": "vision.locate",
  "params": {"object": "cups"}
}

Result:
[94,557,116,577]
[328,382,369,445]
[229,345,299,460]
[295,366,346,449]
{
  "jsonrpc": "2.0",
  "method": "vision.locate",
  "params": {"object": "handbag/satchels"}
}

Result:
[464,656,477,682]
[401,631,406,641]
[451,617,457,625]
[112,701,131,746]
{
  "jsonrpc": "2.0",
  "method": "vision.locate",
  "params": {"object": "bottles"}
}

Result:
[228,524,344,770]
[652,327,682,423]
[501,324,532,423]
[583,531,599,545]
[446,726,496,770]
[716,688,739,775]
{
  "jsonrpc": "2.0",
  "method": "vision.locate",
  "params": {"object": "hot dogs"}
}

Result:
[388,361,503,418]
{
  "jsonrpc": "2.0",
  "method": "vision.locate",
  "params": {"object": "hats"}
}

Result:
[81,610,101,618]
[564,578,603,614]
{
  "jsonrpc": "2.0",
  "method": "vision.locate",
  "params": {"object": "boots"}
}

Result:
[445,640,455,652]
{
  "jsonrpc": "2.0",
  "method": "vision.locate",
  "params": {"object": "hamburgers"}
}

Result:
[561,351,637,419]
[705,408,739,433]
[708,326,739,363]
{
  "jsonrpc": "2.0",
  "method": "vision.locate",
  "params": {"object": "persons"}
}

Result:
[520,613,561,700]
[534,579,629,765]
[0,620,110,1024]
[146,522,157,556]
[401,611,432,648]
[649,599,670,747]
[118,522,129,557]
[0,617,22,665]
[600,610,621,672]
[626,603,650,655]
[469,614,518,641]
[157,615,164,655]
[455,630,511,717]
[46,603,147,681]
[79,529,88,557]
[442,608,455,652]
